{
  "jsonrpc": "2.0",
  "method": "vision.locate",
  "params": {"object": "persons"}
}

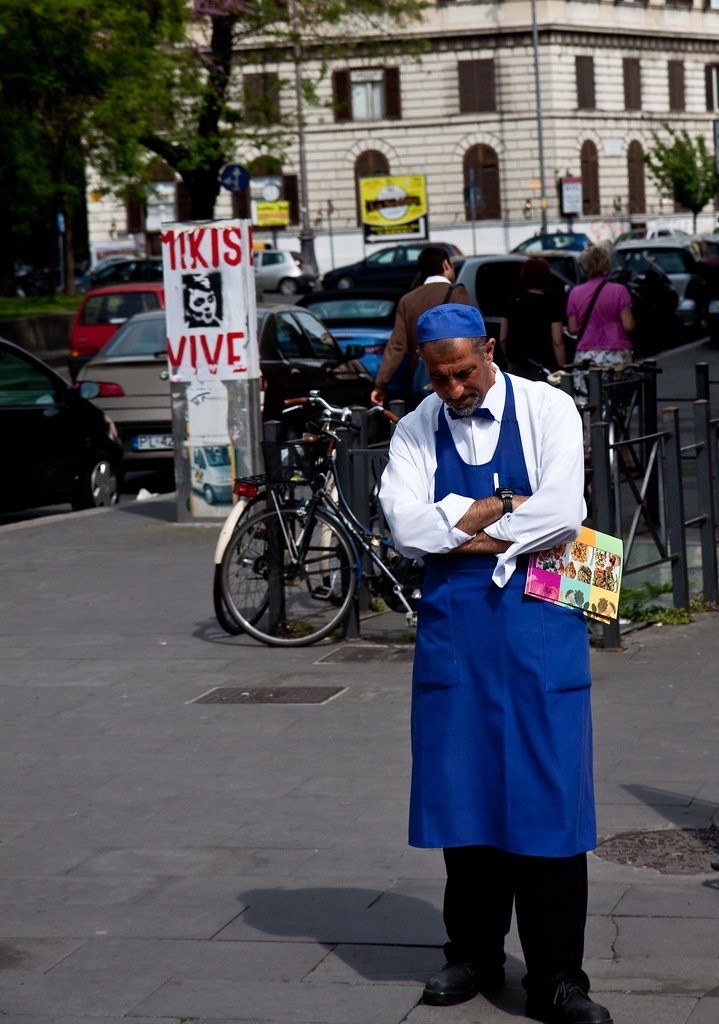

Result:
[567,243,638,463]
[368,246,471,413]
[682,255,719,341]
[498,257,568,387]
[376,302,617,1024]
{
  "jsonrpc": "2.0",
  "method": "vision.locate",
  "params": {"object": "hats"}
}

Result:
[415,303,485,346]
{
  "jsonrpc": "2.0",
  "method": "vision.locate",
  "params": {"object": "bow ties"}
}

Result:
[446,407,494,422]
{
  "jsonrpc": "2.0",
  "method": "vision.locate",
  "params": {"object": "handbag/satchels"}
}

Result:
[412,356,434,397]
[562,328,578,364]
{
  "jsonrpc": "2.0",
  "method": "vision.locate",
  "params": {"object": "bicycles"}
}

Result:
[212,390,397,633]
[222,409,426,648]
[530,357,663,520]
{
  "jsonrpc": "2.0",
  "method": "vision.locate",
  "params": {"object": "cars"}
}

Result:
[74,257,163,298]
[252,229,717,403]
[0,340,126,512]
[77,302,375,472]
[69,284,165,379]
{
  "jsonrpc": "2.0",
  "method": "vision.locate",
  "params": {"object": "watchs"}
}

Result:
[494,487,514,516]
